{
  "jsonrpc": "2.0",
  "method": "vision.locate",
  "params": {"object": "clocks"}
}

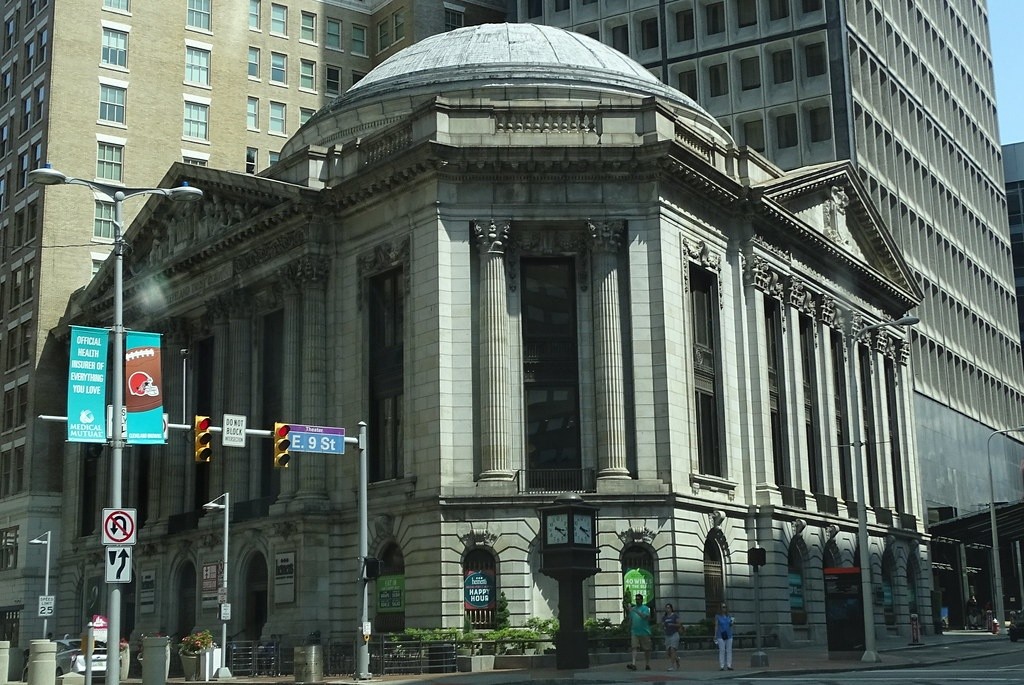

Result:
[546,513,569,545]
[573,514,593,544]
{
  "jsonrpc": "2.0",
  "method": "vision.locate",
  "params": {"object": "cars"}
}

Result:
[23,639,107,683]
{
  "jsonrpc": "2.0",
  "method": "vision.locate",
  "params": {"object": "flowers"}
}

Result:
[181,630,213,655]
[136,632,174,656]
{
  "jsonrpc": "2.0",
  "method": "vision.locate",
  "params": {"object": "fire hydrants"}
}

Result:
[992,618,1000,635]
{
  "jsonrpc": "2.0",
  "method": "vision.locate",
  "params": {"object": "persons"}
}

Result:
[626,594,652,671]
[966,594,981,630]
[714,603,734,670]
[120,638,129,650]
[661,604,681,671]
[127,193,252,277]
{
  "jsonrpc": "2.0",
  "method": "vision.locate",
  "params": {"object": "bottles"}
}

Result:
[628,603,632,611]
[731,616,735,624]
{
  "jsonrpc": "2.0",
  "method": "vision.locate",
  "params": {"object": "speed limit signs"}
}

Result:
[38,595,55,617]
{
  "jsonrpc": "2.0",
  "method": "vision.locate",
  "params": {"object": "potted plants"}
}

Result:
[396,615,776,655]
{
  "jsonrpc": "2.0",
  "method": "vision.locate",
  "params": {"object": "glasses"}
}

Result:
[722,606,727,608]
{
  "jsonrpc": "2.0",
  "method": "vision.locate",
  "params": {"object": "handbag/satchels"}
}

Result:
[677,625,685,636]
[721,631,729,640]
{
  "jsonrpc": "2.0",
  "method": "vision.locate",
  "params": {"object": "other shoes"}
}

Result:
[720,669,724,671]
[667,667,675,671]
[627,664,637,670]
[676,657,681,669]
[646,665,651,671]
[727,667,733,670]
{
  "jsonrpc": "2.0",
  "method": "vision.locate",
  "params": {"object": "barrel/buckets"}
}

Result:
[293,644,324,683]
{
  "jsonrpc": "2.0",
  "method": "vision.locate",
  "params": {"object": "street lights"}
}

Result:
[27,530,51,640]
[851,315,920,663]
[987,427,1024,636]
[26,167,205,685]
[202,492,238,681]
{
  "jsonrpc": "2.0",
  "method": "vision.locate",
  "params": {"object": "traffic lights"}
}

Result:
[366,559,381,579]
[274,421,290,468]
[749,547,766,567]
[195,416,213,463]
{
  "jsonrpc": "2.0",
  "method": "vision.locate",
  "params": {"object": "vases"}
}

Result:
[179,648,197,680]
[137,653,143,666]
